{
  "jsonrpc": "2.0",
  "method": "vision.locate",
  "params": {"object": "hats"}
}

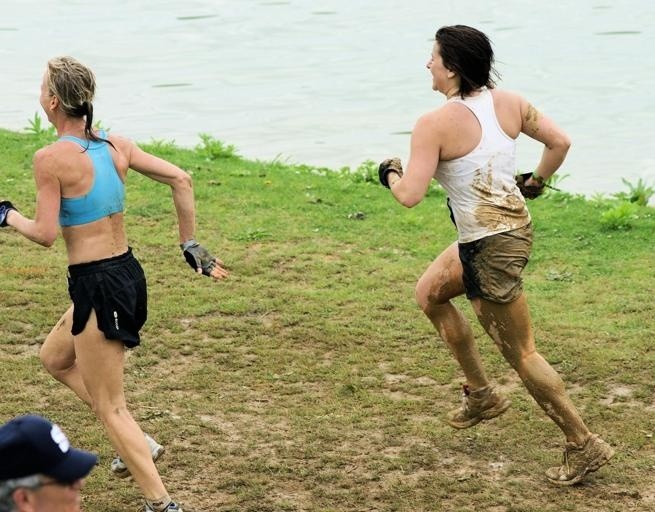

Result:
[0,414,97,486]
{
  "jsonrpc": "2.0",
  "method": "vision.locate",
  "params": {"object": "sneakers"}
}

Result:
[446,381,513,431]
[543,432,616,488]
[111,432,165,479]
[136,501,183,512]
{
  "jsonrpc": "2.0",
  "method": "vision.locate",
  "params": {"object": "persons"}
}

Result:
[378,26,614,487]
[0,414,99,512]
[0,56,229,512]
